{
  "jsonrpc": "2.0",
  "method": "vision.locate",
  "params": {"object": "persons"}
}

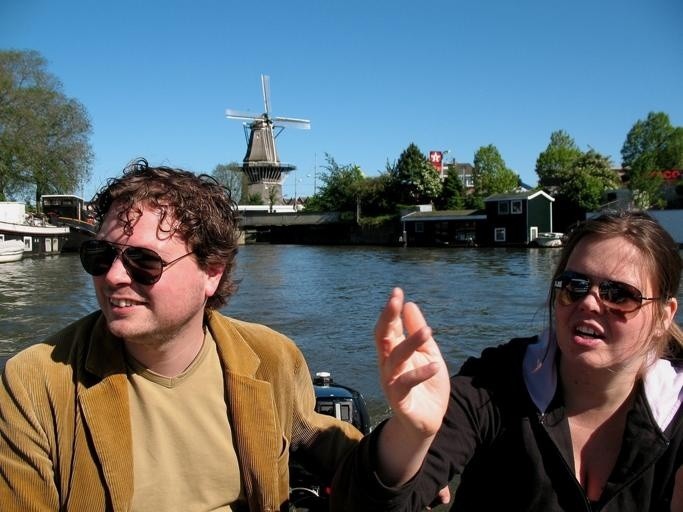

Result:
[337,208,683,510]
[0,154,454,511]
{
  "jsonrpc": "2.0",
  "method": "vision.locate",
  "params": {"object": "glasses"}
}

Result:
[552,270,663,312]
[79,239,196,286]
